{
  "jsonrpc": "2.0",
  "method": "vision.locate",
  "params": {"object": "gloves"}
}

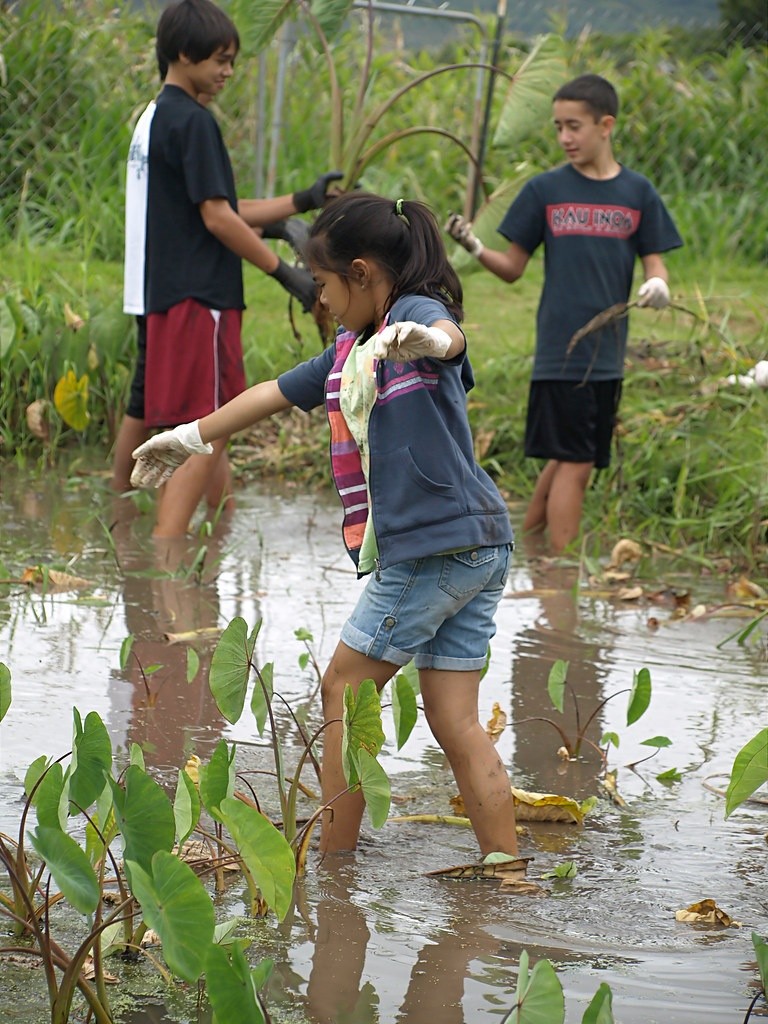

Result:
[374,321,451,362]
[292,174,344,214]
[131,420,214,487]
[443,210,484,256]
[267,256,318,313]
[637,277,671,311]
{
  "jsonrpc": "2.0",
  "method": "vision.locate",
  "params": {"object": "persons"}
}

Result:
[446,74,683,554]
[131,196,514,860]
[115,0,362,508]
